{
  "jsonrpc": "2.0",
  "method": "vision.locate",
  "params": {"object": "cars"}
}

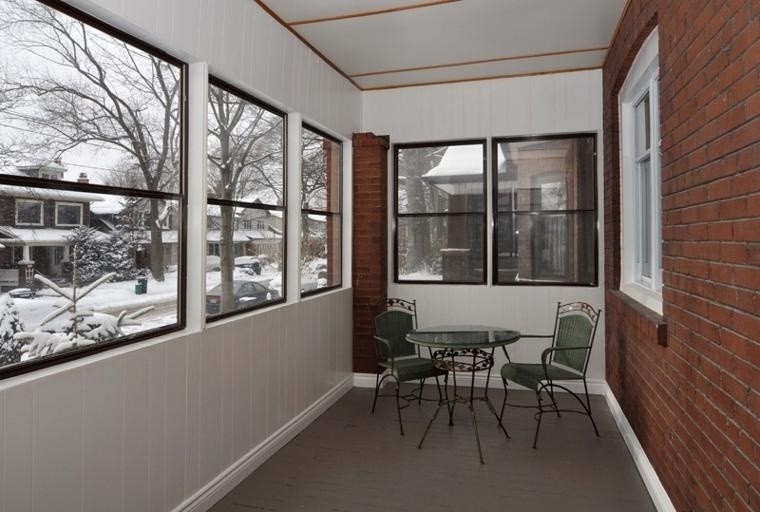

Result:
[206,280,278,314]
[235,256,261,275]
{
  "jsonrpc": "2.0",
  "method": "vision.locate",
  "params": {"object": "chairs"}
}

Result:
[497,302,603,451]
[367,298,456,437]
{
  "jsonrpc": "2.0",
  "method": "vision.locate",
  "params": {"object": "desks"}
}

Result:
[405,324,521,465]
